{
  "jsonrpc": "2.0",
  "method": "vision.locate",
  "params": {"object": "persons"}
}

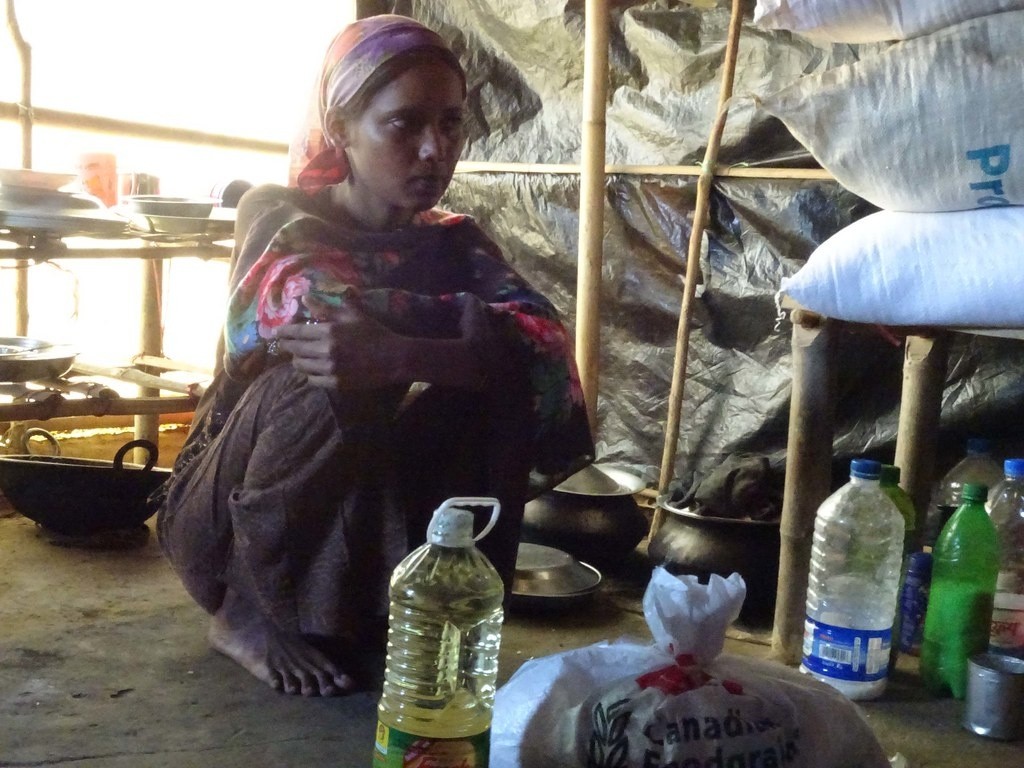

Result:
[157,12,596,698]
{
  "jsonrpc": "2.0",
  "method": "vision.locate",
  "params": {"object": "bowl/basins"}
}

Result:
[113,196,223,218]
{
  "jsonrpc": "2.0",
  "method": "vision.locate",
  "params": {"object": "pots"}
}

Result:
[0,428,174,531]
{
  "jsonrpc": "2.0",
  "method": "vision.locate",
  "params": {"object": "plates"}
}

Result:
[513,542,602,608]
[0,168,80,204]
[0,337,80,383]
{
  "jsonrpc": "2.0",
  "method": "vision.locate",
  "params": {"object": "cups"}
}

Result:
[963,654,1024,740]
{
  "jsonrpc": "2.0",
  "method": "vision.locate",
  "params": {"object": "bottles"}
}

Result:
[921,438,1002,553]
[800,459,905,700]
[372,496,505,768]
[882,465,915,670]
[900,552,932,656]
[983,458,1024,659]
[919,483,1000,699]
